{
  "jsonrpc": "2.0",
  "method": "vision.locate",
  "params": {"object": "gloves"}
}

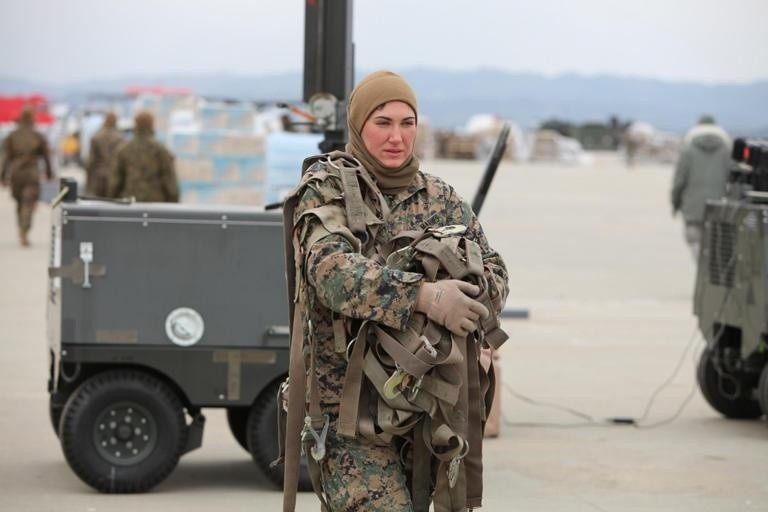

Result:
[417,279,489,339]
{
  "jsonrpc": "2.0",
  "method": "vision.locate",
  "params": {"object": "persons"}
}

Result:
[1,106,54,245]
[671,114,734,275]
[279,72,509,511]
[82,112,125,202]
[103,114,182,203]
